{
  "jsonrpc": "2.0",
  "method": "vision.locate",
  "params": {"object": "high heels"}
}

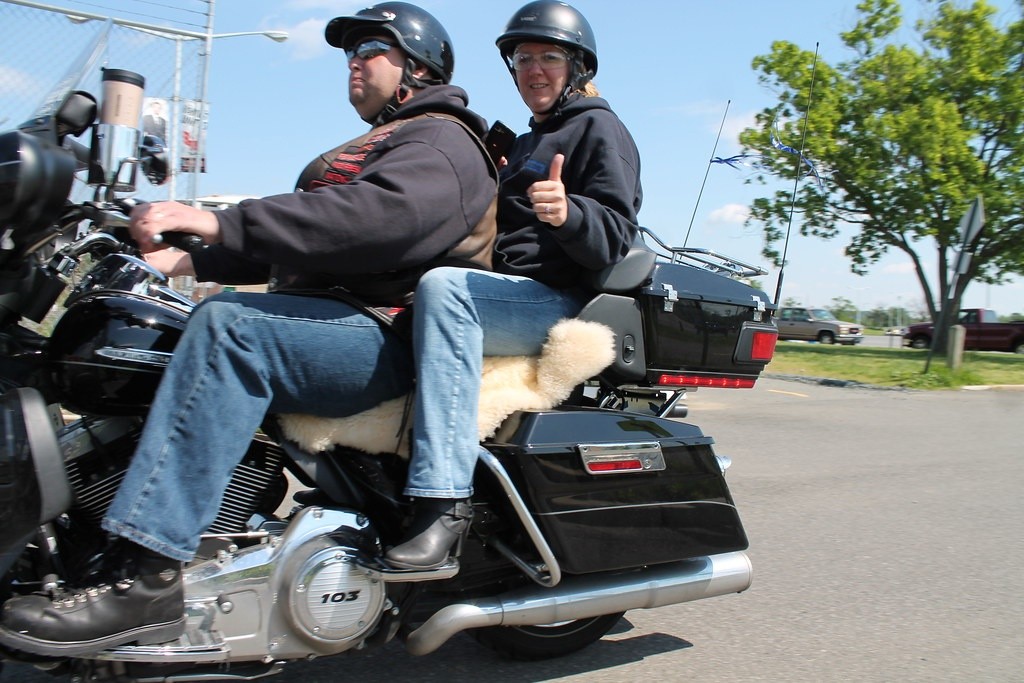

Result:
[294,487,331,508]
[383,497,472,569]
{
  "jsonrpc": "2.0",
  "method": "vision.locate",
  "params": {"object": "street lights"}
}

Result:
[66,12,288,289]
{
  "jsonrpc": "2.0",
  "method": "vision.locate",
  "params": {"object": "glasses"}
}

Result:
[343,38,400,61]
[507,48,576,72]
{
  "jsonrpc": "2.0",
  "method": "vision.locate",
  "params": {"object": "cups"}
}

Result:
[100,67,145,129]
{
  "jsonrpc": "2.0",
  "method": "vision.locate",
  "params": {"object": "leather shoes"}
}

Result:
[0,537,189,656]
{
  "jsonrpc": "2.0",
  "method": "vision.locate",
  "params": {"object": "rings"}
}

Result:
[546,204,549,212]
[155,212,162,217]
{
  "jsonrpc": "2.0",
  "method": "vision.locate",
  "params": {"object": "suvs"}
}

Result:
[778,308,865,346]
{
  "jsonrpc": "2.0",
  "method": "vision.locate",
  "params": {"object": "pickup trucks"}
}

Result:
[902,309,1024,355]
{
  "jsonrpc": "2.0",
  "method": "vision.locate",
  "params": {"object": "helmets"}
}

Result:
[495,0,598,76]
[324,1,456,84]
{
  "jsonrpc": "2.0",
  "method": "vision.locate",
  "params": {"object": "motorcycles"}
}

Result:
[0,90,779,682]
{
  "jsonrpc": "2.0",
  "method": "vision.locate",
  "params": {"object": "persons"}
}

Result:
[379,0,642,570]
[0,0,500,656]
